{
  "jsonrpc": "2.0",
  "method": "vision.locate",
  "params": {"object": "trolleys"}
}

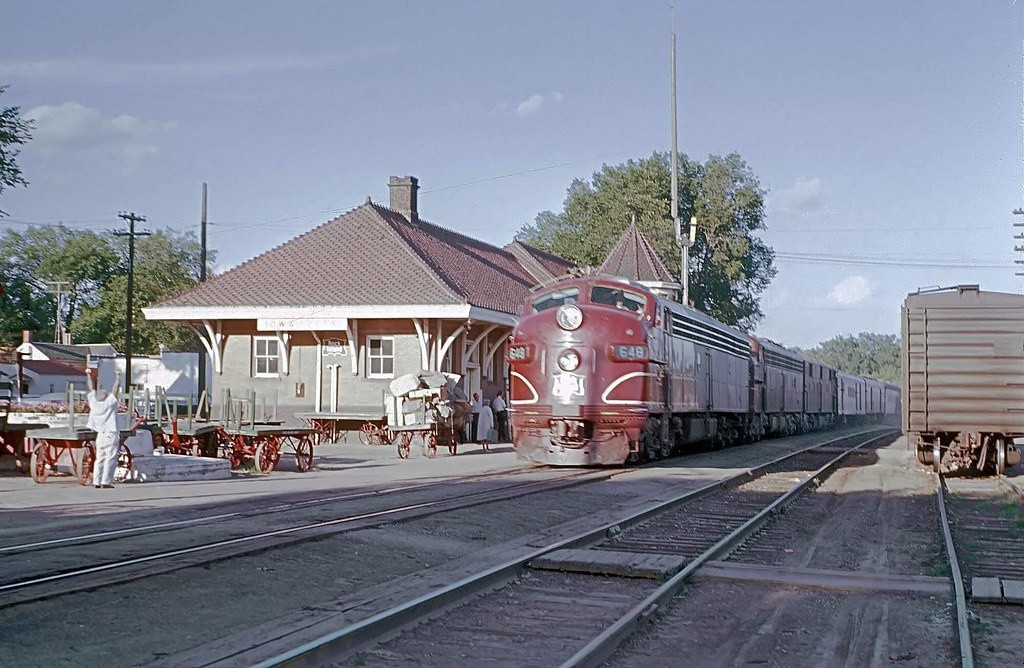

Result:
[383,395,462,458]
[24,382,148,486]
[295,389,392,446]
[120,386,316,479]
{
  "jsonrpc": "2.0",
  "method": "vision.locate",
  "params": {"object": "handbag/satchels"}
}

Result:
[487,428,498,443]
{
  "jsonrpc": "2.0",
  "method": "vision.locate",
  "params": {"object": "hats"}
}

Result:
[95,390,107,402]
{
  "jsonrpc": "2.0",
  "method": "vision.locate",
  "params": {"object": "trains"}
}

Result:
[506,271,901,467]
[900,283,1024,473]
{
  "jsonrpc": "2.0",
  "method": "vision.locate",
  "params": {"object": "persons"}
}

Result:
[476,398,495,453]
[471,393,481,443]
[84,367,121,489]
[492,390,511,444]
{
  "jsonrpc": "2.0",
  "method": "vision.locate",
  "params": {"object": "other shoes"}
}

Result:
[484,449,493,453]
[95,485,100,488]
[102,485,115,488]
